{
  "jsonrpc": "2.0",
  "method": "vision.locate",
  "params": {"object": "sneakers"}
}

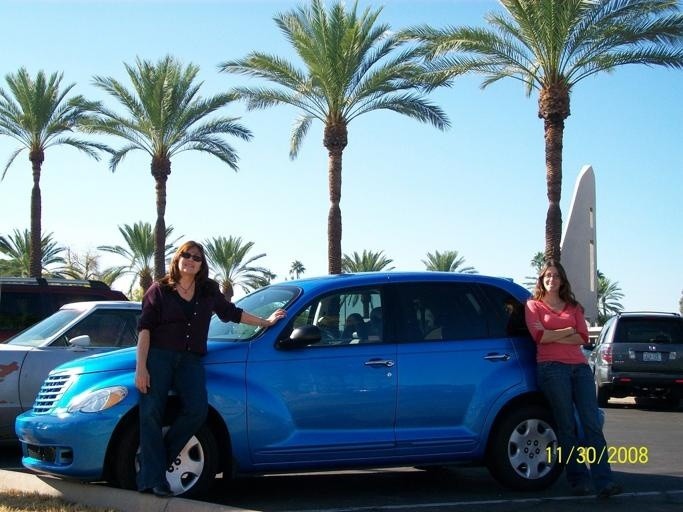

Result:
[570,479,624,501]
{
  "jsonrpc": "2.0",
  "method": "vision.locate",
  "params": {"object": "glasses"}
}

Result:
[181,252,203,262]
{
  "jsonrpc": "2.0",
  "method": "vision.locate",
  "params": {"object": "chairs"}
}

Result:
[342,307,417,343]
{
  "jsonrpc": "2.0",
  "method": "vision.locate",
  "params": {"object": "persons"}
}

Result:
[521,259,625,503]
[132,239,289,499]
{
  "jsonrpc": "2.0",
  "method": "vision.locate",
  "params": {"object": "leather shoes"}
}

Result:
[137,477,174,498]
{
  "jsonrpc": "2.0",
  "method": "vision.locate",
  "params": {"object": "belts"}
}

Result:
[538,361,563,367]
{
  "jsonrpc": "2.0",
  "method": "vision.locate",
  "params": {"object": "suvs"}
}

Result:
[582,311,683,406]
